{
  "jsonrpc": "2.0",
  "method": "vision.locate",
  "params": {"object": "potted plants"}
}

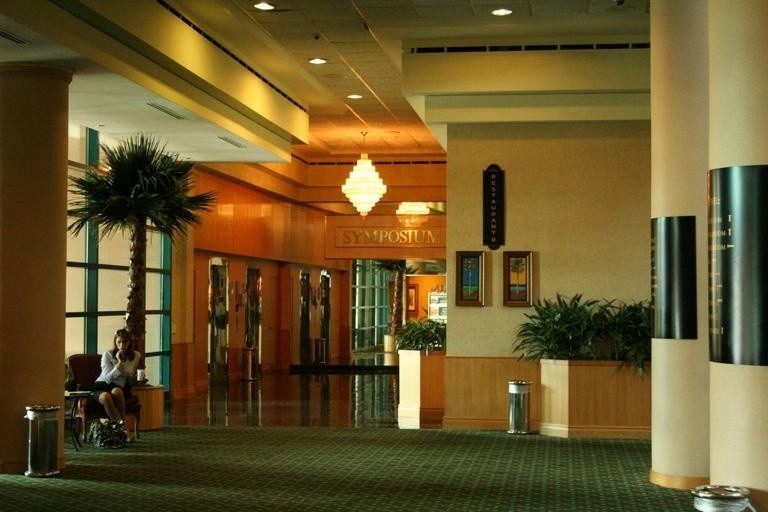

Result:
[65,131,220,431]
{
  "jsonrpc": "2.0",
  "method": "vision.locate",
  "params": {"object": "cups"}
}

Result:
[137,368,145,382]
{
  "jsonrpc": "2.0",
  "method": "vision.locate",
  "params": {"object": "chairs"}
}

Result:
[64,354,142,452]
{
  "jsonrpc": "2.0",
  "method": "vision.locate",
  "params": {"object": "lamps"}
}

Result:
[340,131,388,220]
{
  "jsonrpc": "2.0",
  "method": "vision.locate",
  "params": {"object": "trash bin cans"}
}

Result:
[690,484,751,512]
[25,402,60,477]
[507,380,532,435]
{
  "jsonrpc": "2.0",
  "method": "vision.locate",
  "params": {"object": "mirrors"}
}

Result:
[291,258,446,365]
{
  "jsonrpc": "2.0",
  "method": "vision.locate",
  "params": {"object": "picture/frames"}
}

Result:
[456,251,485,307]
[502,251,532,308]
[407,283,419,318]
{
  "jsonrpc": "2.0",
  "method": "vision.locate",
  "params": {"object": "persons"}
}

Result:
[89,328,142,444]
